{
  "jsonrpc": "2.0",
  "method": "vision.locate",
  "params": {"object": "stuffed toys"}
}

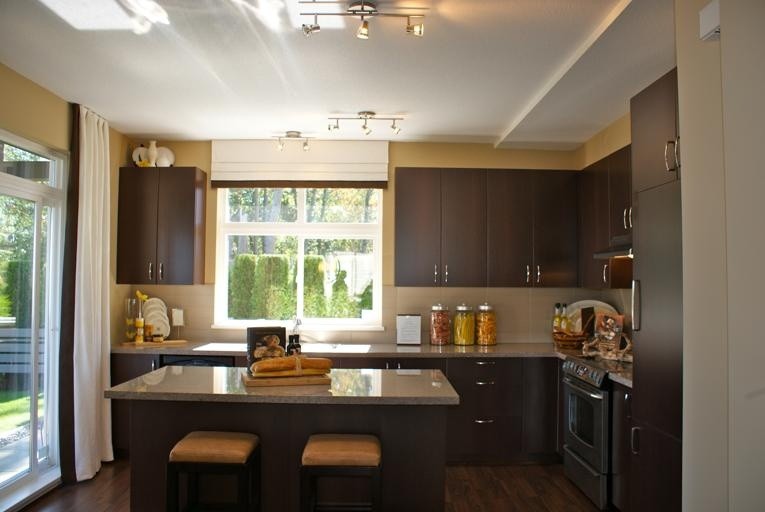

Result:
[252,334,287,360]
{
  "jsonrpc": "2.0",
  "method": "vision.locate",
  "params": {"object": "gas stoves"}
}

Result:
[563,355,632,387]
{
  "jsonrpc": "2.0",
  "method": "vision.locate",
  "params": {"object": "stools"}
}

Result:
[169,430,262,512]
[300,432,382,510]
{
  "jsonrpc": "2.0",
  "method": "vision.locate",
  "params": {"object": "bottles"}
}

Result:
[553,304,562,331]
[287,332,301,356]
[560,304,570,331]
[123,298,138,339]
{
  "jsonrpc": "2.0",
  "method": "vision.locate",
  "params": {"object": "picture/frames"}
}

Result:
[247,325,286,373]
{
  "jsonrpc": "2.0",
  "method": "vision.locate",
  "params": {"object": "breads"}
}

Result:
[250,356,332,372]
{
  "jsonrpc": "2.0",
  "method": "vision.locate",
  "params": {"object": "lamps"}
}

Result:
[273,131,315,152]
[298,1,424,42]
[327,112,404,135]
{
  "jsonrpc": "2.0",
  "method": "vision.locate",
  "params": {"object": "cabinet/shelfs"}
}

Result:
[337,357,444,373]
[117,167,208,287]
[109,354,164,460]
[487,167,579,287]
[626,180,683,511]
[449,360,558,463]
[392,165,486,289]
[611,143,632,254]
[577,157,614,290]
[609,384,629,512]
[630,67,687,193]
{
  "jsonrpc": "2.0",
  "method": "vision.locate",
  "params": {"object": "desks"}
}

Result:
[103,365,463,512]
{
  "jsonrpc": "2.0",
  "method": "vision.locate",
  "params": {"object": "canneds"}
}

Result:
[475,302,497,345]
[454,302,475,346]
[429,303,451,345]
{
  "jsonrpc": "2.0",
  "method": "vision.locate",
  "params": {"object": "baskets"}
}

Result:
[551,331,589,350]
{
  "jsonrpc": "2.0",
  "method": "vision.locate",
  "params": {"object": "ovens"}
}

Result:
[561,374,609,511]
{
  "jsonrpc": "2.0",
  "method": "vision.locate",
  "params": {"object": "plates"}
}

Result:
[567,299,620,336]
[131,146,148,165]
[142,298,172,339]
[156,146,175,166]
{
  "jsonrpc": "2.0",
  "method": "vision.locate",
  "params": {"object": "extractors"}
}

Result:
[591,234,633,259]
